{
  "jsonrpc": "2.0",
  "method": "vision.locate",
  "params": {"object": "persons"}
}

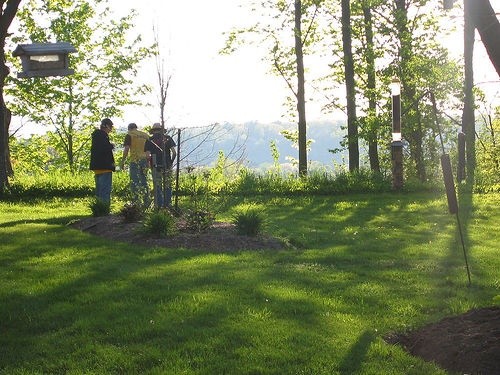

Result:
[121,123,154,209]
[143,122,177,213]
[90,118,118,211]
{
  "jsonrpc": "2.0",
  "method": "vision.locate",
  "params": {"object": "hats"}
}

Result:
[149,123,167,134]
